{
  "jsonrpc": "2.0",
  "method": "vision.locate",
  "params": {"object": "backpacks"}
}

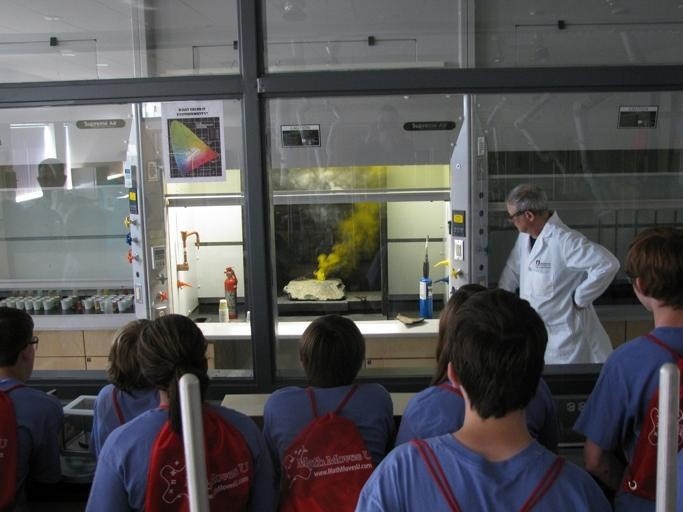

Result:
[0,384,27,512]
[145,402,255,511]
[281,383,374,512]
[621,332,682,503]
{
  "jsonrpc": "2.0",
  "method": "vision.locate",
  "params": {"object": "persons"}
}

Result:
[0,305,64,511]
[90,317,160,463]
[259,313,398,512]
[497,181,622,364]
[393,281,565,453]
[86,314,281,511]
[567,225,683,512]
[353,287,613,512]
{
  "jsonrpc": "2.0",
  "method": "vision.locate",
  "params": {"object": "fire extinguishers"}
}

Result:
[224,267,237,319]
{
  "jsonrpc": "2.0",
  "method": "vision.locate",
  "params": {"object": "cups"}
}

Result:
[0,294,134,313]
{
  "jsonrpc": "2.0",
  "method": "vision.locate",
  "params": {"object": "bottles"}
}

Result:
[218,299,229,323]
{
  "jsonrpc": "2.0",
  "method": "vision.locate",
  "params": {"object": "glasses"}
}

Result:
[506,208,537,223]
[26,335,39,350]
